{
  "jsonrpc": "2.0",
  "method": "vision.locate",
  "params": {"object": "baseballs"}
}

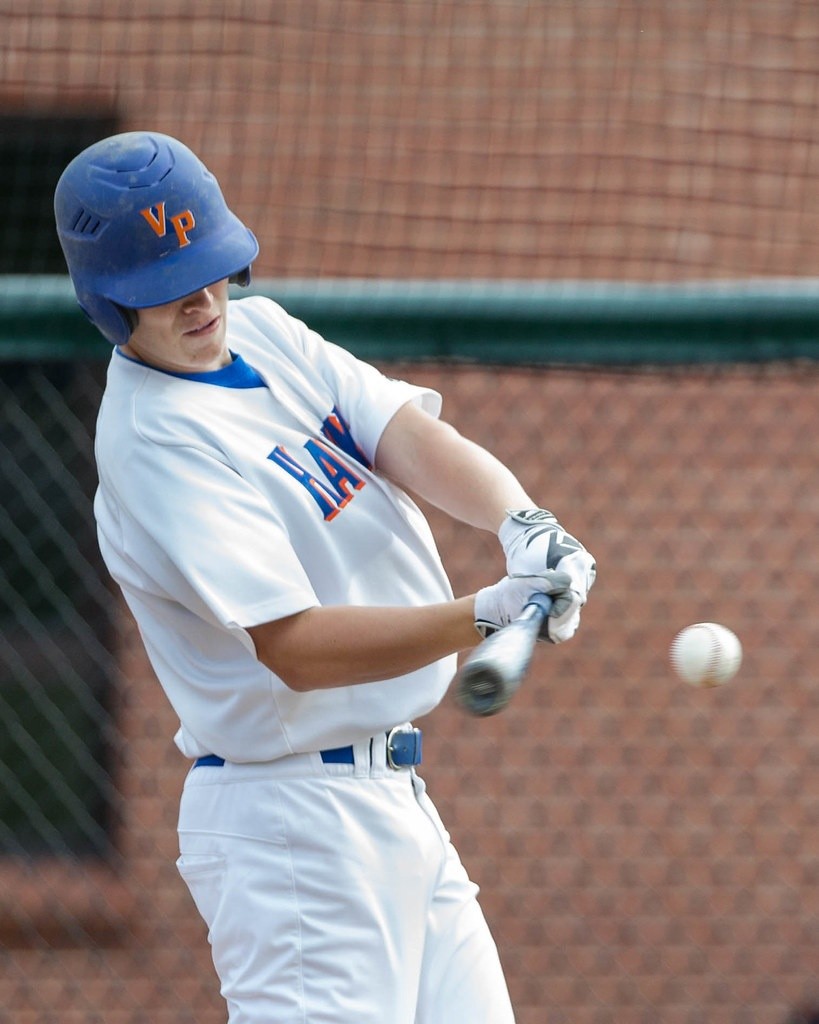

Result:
[669,620,745,688]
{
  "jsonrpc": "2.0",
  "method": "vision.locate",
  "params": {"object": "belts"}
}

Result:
[191,723,422,769]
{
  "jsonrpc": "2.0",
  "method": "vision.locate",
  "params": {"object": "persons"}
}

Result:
[50,129,598,1024]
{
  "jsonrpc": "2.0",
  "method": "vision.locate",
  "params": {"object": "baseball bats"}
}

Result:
[450,594,554,716]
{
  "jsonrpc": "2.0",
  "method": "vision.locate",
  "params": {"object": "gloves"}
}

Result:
[474,571,581,643]
[497,508,597,629]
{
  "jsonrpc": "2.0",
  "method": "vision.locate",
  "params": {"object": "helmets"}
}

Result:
[54,132,259,345]
